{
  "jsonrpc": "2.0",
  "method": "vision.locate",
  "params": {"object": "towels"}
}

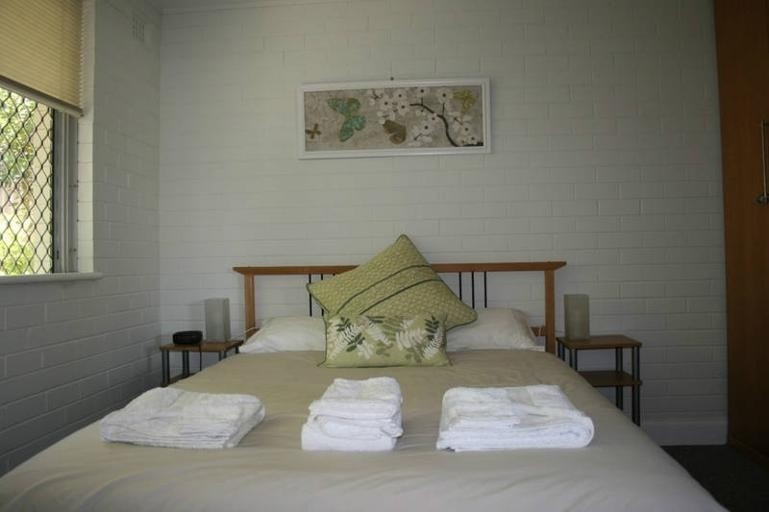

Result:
[303,377,407,452]
[438,387,596,452]
[103,387,264,451]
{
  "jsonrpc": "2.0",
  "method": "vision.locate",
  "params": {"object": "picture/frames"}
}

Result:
[297,76,492,160]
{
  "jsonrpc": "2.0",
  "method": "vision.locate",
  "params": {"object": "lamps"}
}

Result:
[204,297,231,342]
[564,294,591,341]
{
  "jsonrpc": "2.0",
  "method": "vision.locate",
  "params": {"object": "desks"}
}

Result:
[159,341,244,384]
[555,334,642,428]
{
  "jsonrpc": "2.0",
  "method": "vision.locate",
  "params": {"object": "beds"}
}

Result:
[0,260,729,511]
